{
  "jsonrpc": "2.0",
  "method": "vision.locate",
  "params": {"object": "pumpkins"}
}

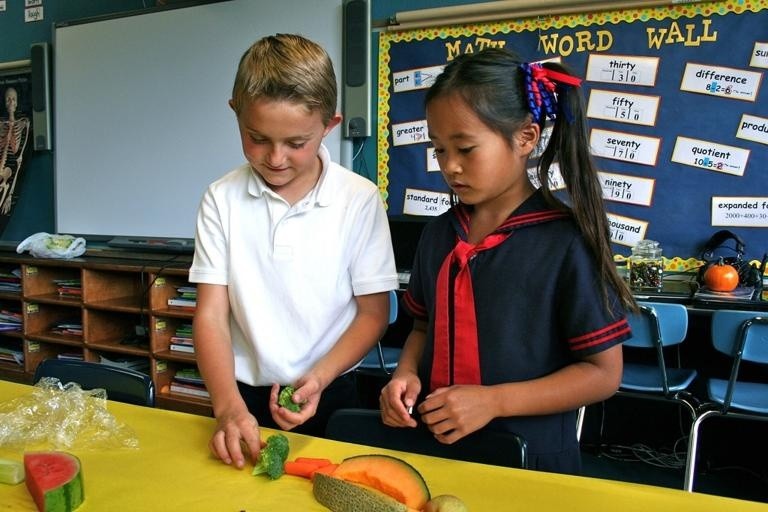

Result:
[705,256,739,292]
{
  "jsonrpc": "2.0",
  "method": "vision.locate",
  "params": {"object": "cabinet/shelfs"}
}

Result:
[0,245,218,421]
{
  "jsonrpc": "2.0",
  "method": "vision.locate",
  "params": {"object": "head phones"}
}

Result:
[696,229,760,286]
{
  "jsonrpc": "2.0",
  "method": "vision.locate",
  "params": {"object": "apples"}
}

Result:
[426,493,469,512]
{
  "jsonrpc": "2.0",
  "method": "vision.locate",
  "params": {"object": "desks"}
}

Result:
[692,286,767,311]
[617,269,696,441]
[0,382,768,512]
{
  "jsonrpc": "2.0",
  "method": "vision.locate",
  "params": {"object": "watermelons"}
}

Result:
[24,449,84,512]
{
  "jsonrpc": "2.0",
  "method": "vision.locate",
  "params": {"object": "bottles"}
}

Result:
[626,239,665,293]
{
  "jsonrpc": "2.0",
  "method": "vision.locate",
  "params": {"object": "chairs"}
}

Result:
[325,408,528,470]
[574,302,699,444]
[344,291,402,409]
[33,357,156,408]
[684,310,768,494]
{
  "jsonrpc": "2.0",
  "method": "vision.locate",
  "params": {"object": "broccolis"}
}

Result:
[278,386,308,412]
[251,448,283,480]
[265,434,290,461]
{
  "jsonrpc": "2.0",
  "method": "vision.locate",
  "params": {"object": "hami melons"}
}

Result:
[313,455,431,512]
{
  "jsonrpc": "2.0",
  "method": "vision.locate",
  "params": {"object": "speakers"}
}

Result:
[342,0,372,139]
[30,41,53,152]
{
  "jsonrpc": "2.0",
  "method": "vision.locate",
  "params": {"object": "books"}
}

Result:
[631,271,768,306]
[0,268,211,398]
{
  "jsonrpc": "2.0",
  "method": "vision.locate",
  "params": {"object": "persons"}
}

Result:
[187,32,402,471]
[377,47,644,475]
